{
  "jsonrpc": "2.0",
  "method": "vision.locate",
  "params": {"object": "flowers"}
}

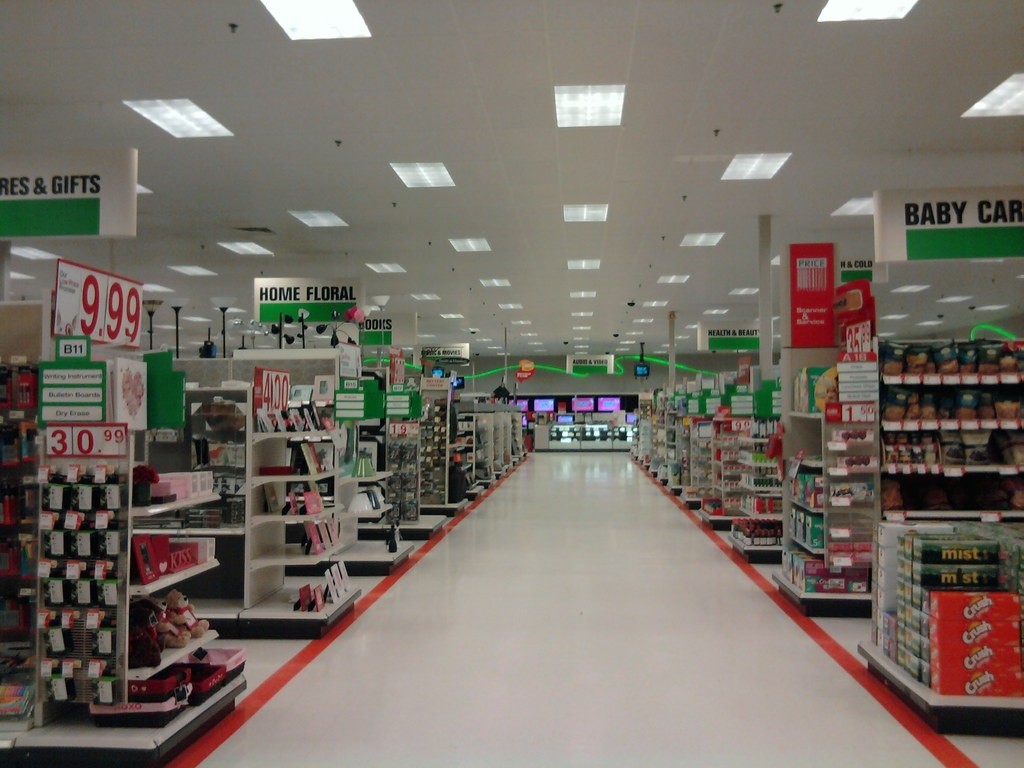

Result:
[133,465,159,484]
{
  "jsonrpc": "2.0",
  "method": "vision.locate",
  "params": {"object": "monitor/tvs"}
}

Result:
[635,364,650,377]
[433,368,445,377]
[452,377,465,389]
[510,398,639,427]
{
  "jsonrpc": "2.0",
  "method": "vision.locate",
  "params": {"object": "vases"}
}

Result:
[134,482,152,507]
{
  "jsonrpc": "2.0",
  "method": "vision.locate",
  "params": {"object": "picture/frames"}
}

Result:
[257,374,350,612]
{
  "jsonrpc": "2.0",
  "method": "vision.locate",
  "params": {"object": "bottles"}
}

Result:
[731,518,783,546]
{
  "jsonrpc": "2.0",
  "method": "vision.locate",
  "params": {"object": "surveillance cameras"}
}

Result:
[564,342,569,344]
[969,306,976,309]
[938,314,943,318]
[471,332,476,335]
[627,302,636,306]
[613,334,619,337]
[476,353,479,356]
[606,352,610,354]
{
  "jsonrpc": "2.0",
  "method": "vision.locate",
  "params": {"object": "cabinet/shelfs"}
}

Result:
[0,345,527,768]
[635,365,1023,616]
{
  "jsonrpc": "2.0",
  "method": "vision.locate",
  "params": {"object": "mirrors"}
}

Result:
[131,535,159,585]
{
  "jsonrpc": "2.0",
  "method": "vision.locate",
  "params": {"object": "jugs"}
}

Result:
[347,482,385,511]
[351,447,375,478]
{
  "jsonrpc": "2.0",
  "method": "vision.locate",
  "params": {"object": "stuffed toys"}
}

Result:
[129,590,210,669]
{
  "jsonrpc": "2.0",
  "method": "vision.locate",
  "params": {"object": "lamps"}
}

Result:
[211,296,356,359]
[168,297,188,358]
[143,299,162,350]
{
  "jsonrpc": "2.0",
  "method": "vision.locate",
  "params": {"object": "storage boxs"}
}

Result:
[88,646,249,726]
[871,519,1024,698]
